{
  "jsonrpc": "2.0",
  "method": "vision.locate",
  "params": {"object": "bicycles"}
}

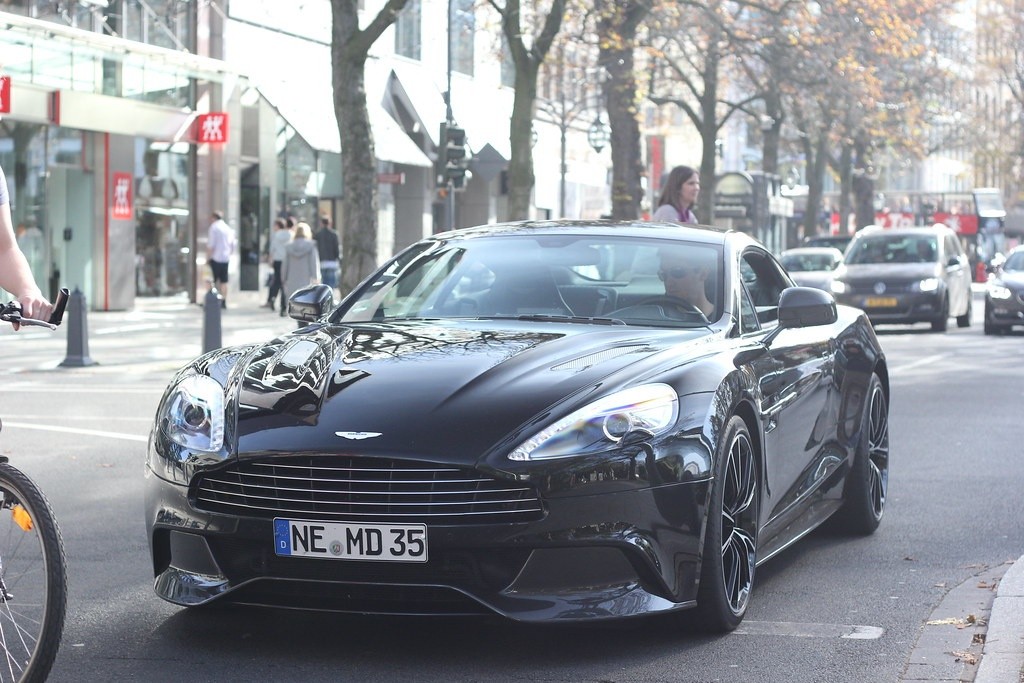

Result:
[0,289,71,683]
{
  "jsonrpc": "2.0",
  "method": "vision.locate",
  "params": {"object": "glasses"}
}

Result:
[658,266,697,281]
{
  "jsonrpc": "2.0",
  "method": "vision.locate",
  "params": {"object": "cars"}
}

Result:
[984,247,1024,337]
[143,218,891,636]
[840,225,971,331]
[769,248,844,299]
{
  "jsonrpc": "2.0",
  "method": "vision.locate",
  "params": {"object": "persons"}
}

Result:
[473,256,556,316]
[263,215,340,329]
[206,213,237,309]
[656,243,744,336]
[0,166,54,330]
[630,165,699,273]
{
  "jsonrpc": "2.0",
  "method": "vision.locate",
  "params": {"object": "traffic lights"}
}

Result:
[438,122,464,188]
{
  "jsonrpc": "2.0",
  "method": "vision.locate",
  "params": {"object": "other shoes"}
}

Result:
[282,308,288,316]
[260,301,272,307]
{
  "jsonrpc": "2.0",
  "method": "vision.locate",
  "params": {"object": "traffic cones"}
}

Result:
[57,289,101,367]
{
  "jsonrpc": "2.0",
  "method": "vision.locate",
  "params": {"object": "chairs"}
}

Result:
[480,265,572,316]
